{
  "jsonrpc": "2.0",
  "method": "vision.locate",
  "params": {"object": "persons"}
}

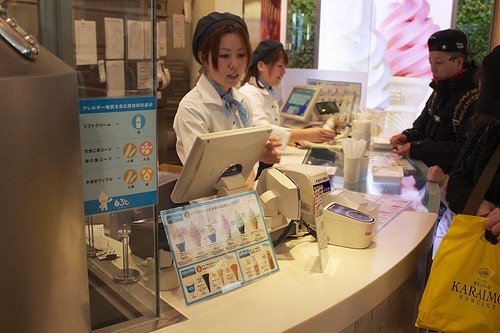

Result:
[172,11,282,184]
[238,39,338,148]
[427,45,500,213]
[477,192,500,240]
[389,29,482,177]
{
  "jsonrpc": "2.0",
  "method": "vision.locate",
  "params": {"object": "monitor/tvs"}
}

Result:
[171,126,273,204]
[279,84,323,122]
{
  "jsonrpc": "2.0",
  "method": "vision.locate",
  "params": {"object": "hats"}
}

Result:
[193,12,247,65]
[249,39,284,68]
[428,29,477,55]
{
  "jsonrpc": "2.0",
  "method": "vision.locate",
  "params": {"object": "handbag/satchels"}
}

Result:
[415,214,500,333]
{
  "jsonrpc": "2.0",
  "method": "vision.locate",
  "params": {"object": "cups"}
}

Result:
[360,156,369,176]
[343,157,361,184]
